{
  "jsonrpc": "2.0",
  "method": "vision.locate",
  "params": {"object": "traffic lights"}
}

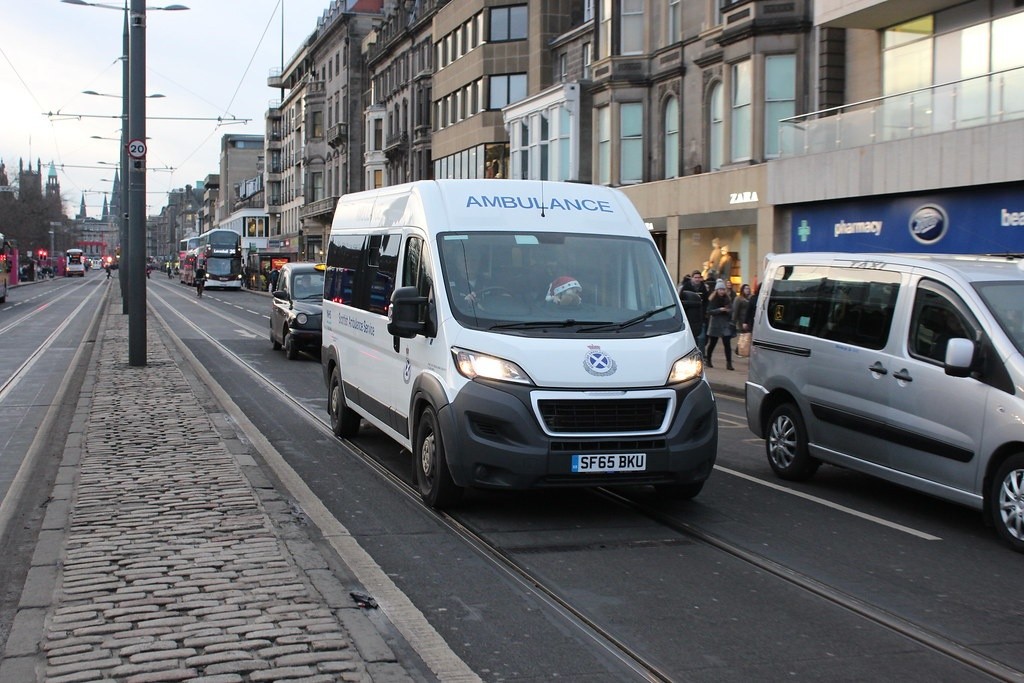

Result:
[39,250,43,260]
[43,251,47,260]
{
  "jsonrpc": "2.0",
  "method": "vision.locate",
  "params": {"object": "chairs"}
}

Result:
[919,309,950,357]
[529,257,574,300]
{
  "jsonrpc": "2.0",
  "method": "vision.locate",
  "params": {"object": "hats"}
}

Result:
[715,279,726,289]
[712,237,720,243]
[721,245,728,251]
[545,276,582,306]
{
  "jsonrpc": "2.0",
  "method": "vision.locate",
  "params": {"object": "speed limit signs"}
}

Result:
[127,139,147,159]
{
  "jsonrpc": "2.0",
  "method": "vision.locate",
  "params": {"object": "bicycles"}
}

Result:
[194,276,208,299]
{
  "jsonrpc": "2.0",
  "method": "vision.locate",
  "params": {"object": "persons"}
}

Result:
[724,279,737,303]
[705,279,736,370]
[195,266,206,296]
[297,274,318,293]
[679,270,709,357]
[732,284,752,355]
[427,244,513,330]
[105,265,110,279]
[742,282,762,331]
[675,270,716,303]
[167,267,171,278]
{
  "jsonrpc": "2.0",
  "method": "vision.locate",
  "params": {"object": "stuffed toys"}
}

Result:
[546,277,583,306]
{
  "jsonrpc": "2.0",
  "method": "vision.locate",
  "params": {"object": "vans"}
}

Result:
[319,178,719,515]
[742,253,1024,553]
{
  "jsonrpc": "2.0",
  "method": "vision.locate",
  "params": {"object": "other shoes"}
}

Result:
[726,359,734,370]
[706,357,713,367]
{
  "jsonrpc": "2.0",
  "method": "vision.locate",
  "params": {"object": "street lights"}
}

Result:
[60,0,192,368]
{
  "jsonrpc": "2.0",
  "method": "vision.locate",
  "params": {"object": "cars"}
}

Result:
[269,260,321,360]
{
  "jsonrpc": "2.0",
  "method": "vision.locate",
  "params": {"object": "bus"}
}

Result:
[178,227,243,292]
[65,248,85,277]
[0,232,11,304]
